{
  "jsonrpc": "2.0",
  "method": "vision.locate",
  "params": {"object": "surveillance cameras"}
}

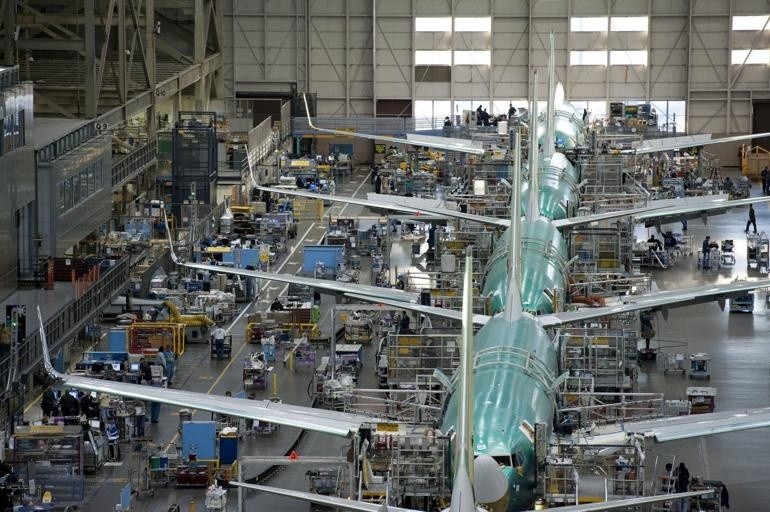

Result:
[156,21,161,34]
[14,26,20,41]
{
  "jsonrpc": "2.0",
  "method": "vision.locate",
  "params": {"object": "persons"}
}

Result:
[270,287,410,334]
[647,165,769,270]
[444,103,516,138]
[43,343,257,512]
[271,194,293,211]
[296,161,381,194]
[582,108,589,134]
[673,461,690,508]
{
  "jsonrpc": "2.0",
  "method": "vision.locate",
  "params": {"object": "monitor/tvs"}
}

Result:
[316,155,335,161]
[23,361,145,429]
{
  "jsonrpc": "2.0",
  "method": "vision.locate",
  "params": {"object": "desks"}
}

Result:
[685,386,717,414]
